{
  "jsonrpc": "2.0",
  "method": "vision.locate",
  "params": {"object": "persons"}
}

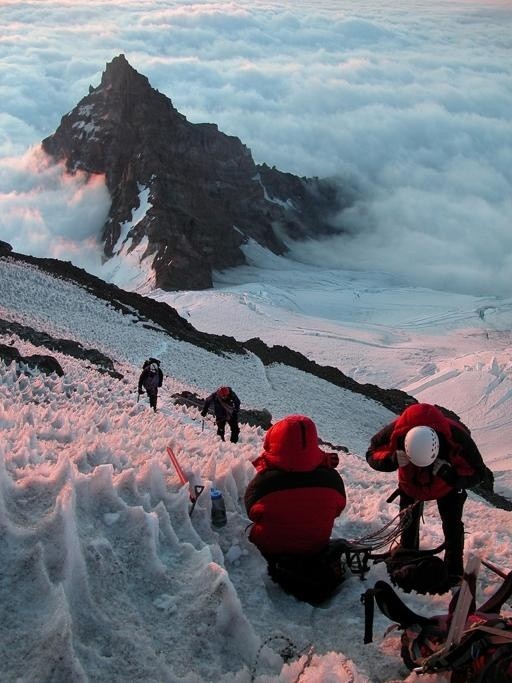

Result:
[367,403,512,589]
[138,361,163,412]
[203,386,240,443]
[244,416,345,571]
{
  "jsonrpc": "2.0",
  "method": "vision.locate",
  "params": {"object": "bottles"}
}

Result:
[208,486,228,528]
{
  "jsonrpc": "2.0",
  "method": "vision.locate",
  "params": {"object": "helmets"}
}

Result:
[404,426,439,468]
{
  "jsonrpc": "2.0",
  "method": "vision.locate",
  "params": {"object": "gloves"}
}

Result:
[432,457,457,480]
[389,449,410,470]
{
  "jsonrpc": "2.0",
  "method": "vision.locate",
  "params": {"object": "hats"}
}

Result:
[150,362,159,371]
[217,386,230,397]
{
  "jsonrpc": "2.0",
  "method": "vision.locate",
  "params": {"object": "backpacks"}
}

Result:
[383,541,462,596]
[268,538,373,608]
[359,554,512,682]
[142,357,161,370]
